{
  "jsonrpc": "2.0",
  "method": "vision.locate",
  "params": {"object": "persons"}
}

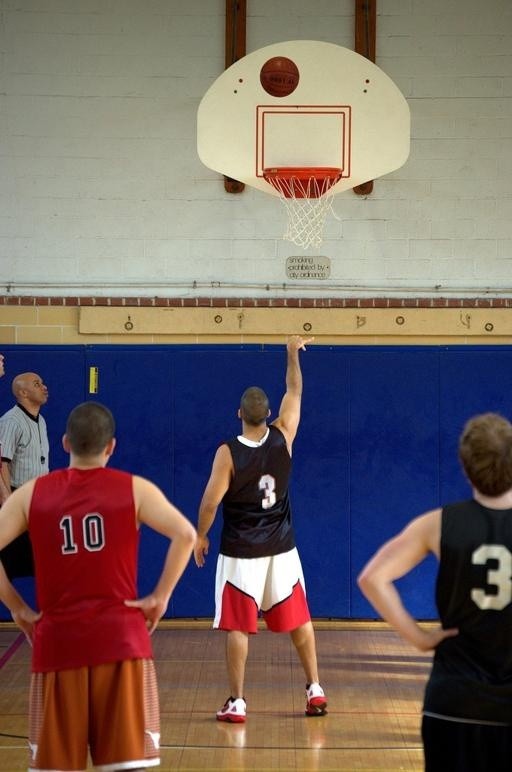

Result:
[0,371,51,580]
[194,336,327,721]
[357,412,511,772]
[0,353,6,378]
[0,400,196,772]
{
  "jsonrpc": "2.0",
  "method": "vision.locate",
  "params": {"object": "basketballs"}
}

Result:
[260,56,299,97]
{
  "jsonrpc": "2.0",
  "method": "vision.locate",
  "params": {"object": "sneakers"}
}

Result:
[216,696,247,723]
[304,682,327,716]
